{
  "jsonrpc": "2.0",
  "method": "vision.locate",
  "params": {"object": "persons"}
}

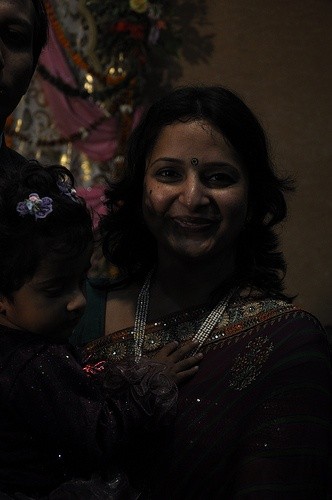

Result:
[0,144,205,500]
[0,0,52,166]
[48,83,332,499]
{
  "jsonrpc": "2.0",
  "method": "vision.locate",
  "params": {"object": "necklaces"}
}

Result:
[134,273,243,370]
